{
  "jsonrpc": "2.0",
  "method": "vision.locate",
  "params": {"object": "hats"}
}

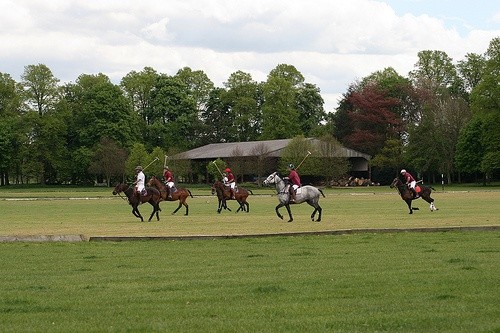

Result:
[400,169,406,173]
[225,167,231,172]
[289,164,295,169]
[163,166,169,170]
[135,165,142,171]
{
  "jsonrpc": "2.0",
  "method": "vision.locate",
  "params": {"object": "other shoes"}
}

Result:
[231,196,235,199]
[413,195,417,199]
[290,198,296,203]
[138,199,142,205]
[168,194,173,198]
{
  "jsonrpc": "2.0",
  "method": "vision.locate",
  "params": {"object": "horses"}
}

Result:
[390,177,439,214]
[263,172,326,223]
[113,176,194,222]
[211,180,254,213]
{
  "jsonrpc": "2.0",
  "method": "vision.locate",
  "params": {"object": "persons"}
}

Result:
[283,164,301,201]
[401,170,419,198]
[161,166,174,198]
[132,166,146,205]
[221,168,236,199]
